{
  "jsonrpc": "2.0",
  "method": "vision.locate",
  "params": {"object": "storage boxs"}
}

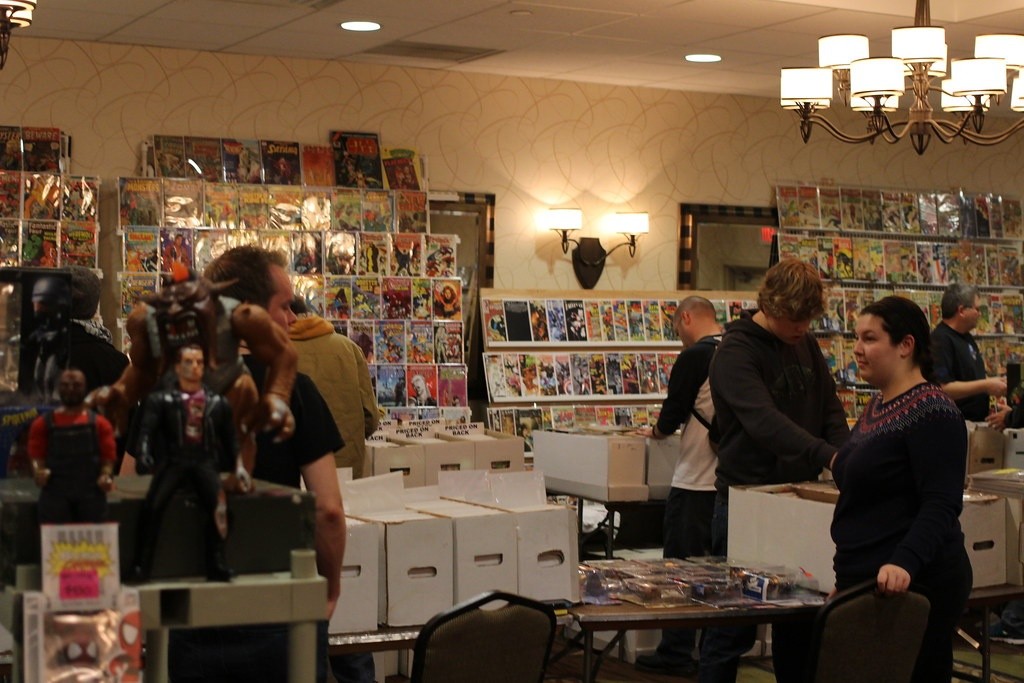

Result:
[961,488,1006,590]
[530,430,647,502]
[1003,427,1024,469]
[645,433,683,502]
[438,486,583,608]
[439,424,529,473]
[388,430,475,483]
[362,434,424,488]
[399,496,518,613]
[344,503,453,630]
[723,482,836,595]
[327,513,380,637]
[966,424,1006,475]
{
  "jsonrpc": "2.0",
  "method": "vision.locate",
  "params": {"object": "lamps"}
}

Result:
[778,0,1024,157]
[543,209,650,289]
[0,0,37,71]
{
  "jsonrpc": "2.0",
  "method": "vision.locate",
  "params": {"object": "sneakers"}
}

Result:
[636,650,695,677]
[980,620,1023,646]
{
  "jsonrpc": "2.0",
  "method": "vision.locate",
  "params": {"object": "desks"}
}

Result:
[327,554,1024,683]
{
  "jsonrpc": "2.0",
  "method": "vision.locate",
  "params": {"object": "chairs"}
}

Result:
[782,570,934,683]
[385,585,560,683]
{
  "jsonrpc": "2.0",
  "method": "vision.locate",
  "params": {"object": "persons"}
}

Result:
[286,297,380,682]
[28,367,116,523]
[986,403,1024,433]
[126,269,298,492]
[35,266,130,400]
[928,283,1006,423]
[827,295,973,683]
[635,295,728,674]
[204,244,347,683]
[698,260,851,683]
[140,344,252,581]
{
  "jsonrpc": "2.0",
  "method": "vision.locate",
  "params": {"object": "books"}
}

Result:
[0,125,98,267]
[481,296,757,458]
[776,184,1023,420]
[117,130,472,422]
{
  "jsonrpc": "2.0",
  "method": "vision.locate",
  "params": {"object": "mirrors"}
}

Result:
[678,202,778,292]
[430,190,496,403]
